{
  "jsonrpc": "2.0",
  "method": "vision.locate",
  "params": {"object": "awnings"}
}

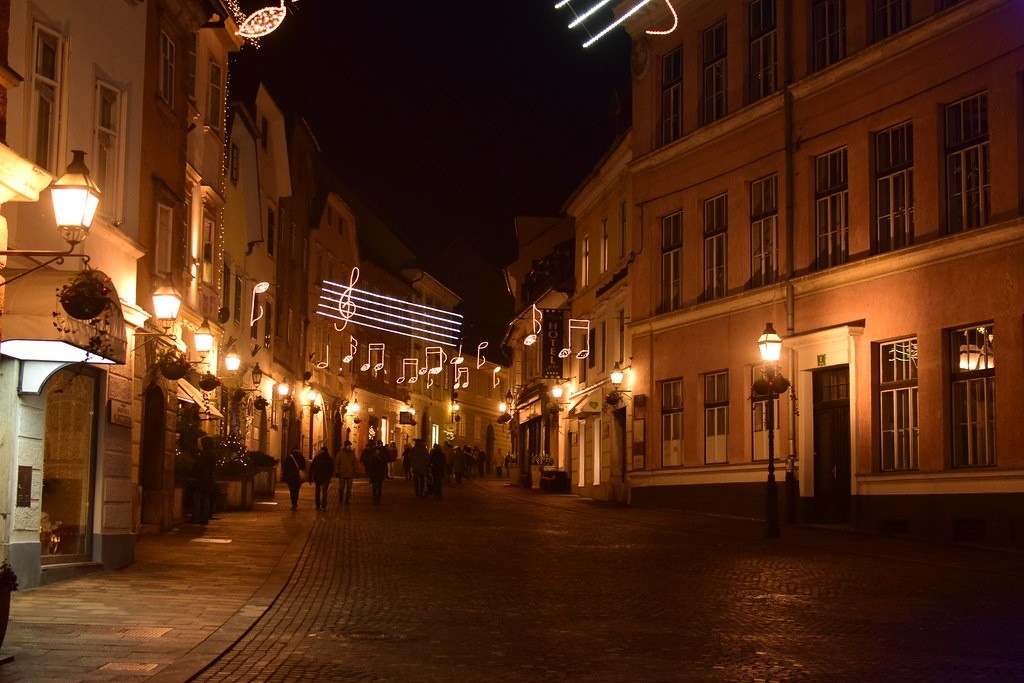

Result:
[178,378,224,420]
[0,269,126,397]
[562,388,602,419]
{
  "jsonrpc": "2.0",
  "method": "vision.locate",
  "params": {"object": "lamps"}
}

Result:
[243,362,263,392]
[189,317,214,369]
[278,376,289,396]
[611,362,631,393]
[219,344,241,379]
[130,272,183,355]
[506,388,518,410]
[0,150,102,287]
[552,379,570,404]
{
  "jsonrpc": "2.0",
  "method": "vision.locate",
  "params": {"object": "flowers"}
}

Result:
[198,374,221,414]
[52,264,123,393]
[145,345,195,378]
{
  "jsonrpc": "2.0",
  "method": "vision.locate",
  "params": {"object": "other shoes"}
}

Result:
[322,505,325,511]
[291,505,298,511]
[316,507,321,513]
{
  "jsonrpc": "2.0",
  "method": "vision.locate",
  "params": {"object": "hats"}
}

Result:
[345,440,352,448]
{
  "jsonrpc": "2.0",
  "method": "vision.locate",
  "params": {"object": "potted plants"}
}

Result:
[0,557,19,649]
[751,371,800,429]
[215,451,280,511]
[604,390,623,408]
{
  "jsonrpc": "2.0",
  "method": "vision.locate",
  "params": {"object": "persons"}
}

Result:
[308,446,334,508]
[282,444,306,510]
[493,448,511,478]
[335,439,358,502]
[360,439,398,496]
[401,438,486,499]
[188,437,216,525]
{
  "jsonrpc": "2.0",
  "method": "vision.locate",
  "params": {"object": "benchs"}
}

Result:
[540,471,566,493]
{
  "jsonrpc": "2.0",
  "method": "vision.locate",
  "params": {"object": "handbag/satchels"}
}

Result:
[299,470,307,481]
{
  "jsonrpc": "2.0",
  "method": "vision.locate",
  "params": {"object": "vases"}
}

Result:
[199,381,217,391]
[161,364,186,380]
[61,297,105,319]
